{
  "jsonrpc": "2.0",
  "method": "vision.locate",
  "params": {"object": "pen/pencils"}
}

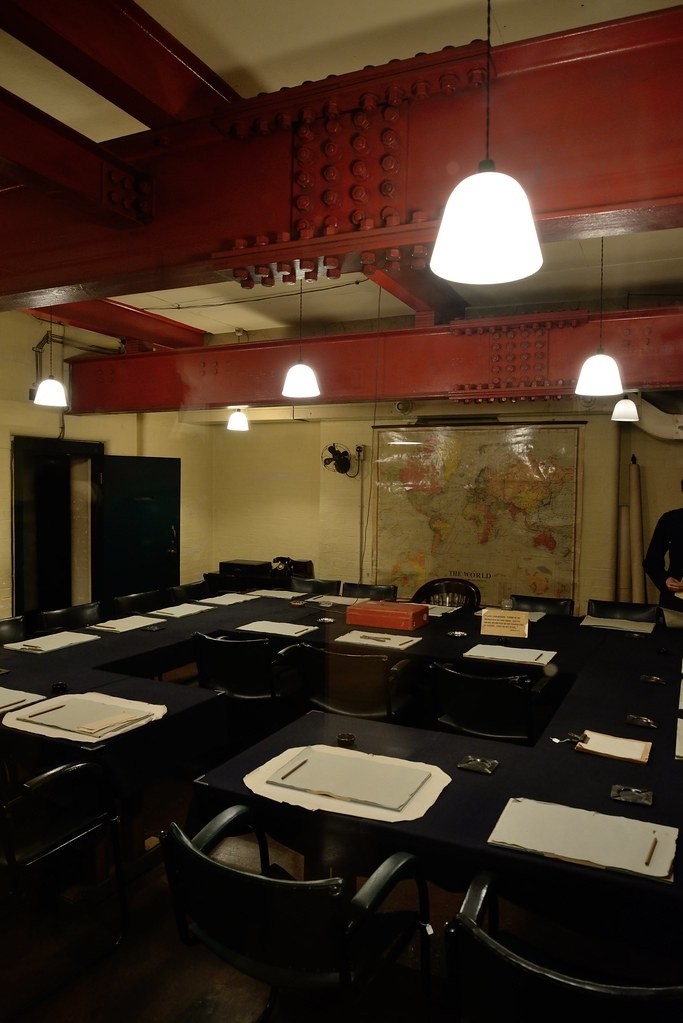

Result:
[361,635,391,642]
[281,759,308,779]
[399,640,413,646]
[95,625,116,629]
[29,704,65,717]
[645,837,657,865]
[21,648,43,651]
[295,629,309,634]
[157,611,174,615]
[23,644,40,648]
[0,699,26,709]
[535,653,543,661]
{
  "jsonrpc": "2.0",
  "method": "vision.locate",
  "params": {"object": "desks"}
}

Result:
[0,587,683,901]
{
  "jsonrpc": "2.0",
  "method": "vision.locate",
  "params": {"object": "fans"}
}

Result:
[322,444,365,479]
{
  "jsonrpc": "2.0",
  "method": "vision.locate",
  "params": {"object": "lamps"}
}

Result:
[282,268,320,398]
[227,406,251,431]
[32,302,67,406]
[431,1,546,285]
[612,396,638,422]
[574,229,623,398]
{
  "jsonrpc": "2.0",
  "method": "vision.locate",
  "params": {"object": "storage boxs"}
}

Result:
[344,601,429,630]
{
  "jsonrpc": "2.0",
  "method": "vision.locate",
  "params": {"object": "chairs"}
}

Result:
[1,756,683,1023]
[0,579,666,745]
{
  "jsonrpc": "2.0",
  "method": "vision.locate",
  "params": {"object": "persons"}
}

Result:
[643,479,683,615]
